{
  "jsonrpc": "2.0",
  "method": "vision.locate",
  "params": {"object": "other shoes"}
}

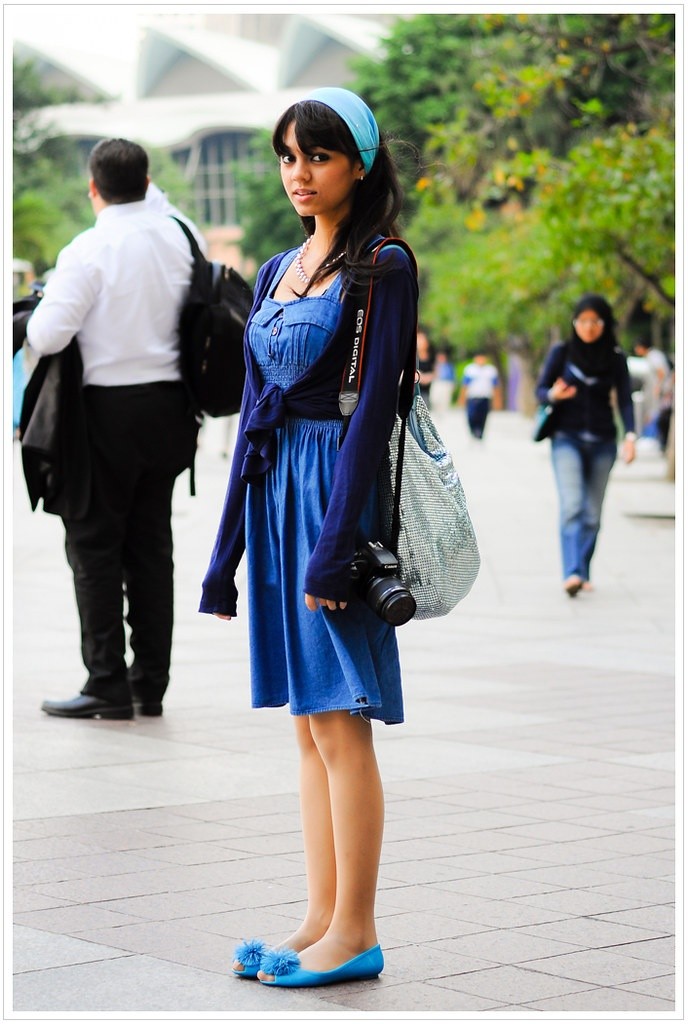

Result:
[584,583,592,591]
[563,576,581,596]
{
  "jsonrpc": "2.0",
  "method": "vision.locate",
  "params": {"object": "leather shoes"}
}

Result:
[42,694,134,720]
[131,697,163,717]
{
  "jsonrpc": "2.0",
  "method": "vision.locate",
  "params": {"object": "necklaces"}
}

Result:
[295,235,346,284]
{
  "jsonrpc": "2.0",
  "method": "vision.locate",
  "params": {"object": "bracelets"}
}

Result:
[625,431,637,442]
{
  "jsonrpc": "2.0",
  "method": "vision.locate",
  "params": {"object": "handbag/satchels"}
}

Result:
[353,235,482,621]
[531,343,573,442]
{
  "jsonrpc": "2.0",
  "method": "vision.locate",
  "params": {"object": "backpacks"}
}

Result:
[167,213,254,418]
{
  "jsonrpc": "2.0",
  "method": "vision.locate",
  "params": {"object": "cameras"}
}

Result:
[342,536,417,626]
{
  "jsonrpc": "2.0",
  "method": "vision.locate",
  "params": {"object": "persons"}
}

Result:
[535,294,637,596]
[26,138,207,720]
[413,329,675,482]
[199,87,419,986]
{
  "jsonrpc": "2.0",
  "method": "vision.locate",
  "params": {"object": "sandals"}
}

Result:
[231,939,274,977]
[257,943,384,989]
